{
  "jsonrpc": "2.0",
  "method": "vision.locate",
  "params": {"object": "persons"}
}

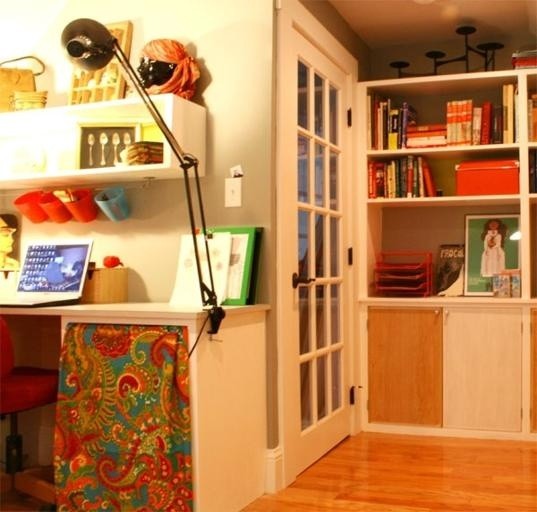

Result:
[138,38,201,98]
[480,219,507,292]
[0,217,19,270]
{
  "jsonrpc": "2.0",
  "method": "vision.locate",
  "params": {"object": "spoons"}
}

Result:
[111,130,120,166]
[98,131,109,167]
[122,132,132,150]
[86,132,96,167]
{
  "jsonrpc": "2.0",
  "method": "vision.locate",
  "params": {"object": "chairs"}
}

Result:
[1,315,58,500]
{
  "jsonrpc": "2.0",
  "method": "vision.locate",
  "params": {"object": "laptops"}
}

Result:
[0,239,93,307]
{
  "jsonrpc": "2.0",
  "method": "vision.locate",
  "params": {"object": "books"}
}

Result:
[436,244,464,295]
[368,84,536,199]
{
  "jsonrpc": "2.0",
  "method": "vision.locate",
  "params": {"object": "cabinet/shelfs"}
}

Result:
[358,68,537,438]
[0,91,207,189]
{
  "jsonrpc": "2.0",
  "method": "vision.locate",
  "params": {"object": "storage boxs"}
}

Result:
[455,160,519,196]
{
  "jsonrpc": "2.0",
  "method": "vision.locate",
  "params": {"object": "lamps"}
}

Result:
[61,19,224,338]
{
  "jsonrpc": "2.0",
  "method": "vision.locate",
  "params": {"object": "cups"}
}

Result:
[38,192,73,223]
[93,187,132,223]
[60,189,98,222]
[13,190,50,224]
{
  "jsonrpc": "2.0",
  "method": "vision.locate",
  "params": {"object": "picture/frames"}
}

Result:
[465,213,520,296]
[76,123,143,170]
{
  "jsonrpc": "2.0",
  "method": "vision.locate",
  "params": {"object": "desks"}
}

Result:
[0,300,271,512]
